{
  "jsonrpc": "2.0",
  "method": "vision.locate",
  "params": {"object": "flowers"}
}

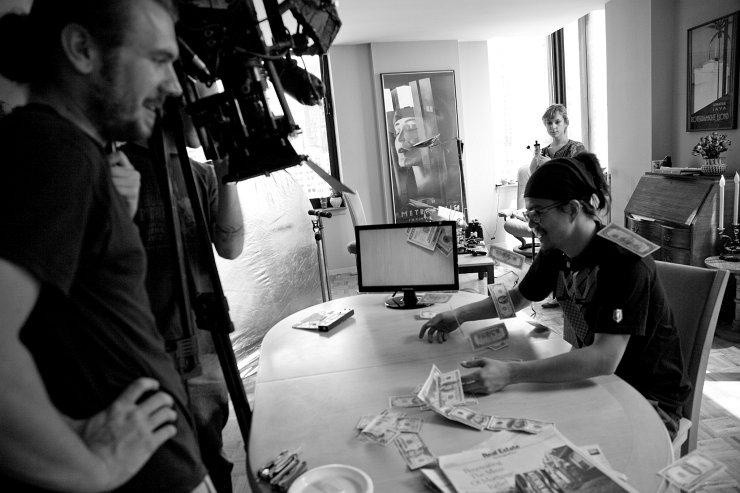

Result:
[690,133,735,162]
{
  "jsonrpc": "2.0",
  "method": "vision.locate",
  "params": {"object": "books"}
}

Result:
[418,423,640,493]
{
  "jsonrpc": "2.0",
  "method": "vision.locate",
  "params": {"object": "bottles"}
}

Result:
[541,148,550,157]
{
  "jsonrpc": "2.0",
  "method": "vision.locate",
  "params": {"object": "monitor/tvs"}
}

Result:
[355,220,459,309]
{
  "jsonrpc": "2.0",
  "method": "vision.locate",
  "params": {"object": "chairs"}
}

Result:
[626,259,731,493]
[498,167,542,259]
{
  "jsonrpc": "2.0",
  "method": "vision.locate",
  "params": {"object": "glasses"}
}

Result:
[522,198,572,224]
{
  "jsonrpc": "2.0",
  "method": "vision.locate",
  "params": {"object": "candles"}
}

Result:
[719,174,725,229]
[733,171,740,225]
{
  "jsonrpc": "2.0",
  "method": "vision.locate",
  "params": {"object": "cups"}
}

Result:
[320,197,328,210]
[651,159,665,173]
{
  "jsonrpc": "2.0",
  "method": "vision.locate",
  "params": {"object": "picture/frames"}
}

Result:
[380,69,463,228]
[686,9,740,132]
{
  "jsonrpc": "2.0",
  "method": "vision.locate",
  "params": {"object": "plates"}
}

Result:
[286,464,374,493]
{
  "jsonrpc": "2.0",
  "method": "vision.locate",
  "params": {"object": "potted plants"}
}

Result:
[329,187,343,209]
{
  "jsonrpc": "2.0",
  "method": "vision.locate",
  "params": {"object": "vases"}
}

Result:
[700,158,729,177]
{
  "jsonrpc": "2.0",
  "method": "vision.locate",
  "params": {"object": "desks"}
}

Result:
[245,288,675,493]
[704,255,740,343]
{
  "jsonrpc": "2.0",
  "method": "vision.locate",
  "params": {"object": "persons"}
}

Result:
[117,70,247,493]
[393,79,458,190]
[528,102,589,174]
[1,0,222,493]
[417,150,692,444]
